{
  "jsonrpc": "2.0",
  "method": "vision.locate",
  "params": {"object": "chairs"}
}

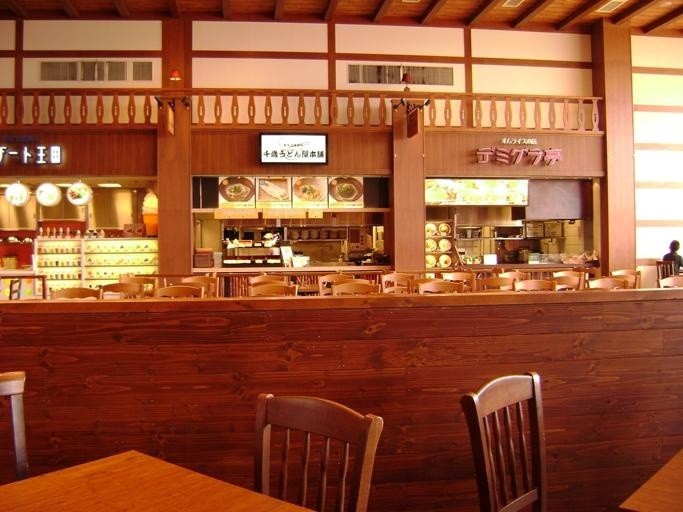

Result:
[253,389,386,511]
[462,369,554,512]
[0,260,682,304]
[0,366,33,482]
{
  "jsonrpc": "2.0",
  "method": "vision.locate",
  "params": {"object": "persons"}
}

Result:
[662,241,683,281]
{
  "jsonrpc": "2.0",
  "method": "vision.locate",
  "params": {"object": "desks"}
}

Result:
[618,447,682,512]
[0,448,312,512]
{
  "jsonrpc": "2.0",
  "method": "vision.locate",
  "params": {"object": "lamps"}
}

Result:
[169,69,181,87]
[401,73,412,91]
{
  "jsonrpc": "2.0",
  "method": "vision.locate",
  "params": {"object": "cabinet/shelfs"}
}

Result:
[34,234,158,289]
